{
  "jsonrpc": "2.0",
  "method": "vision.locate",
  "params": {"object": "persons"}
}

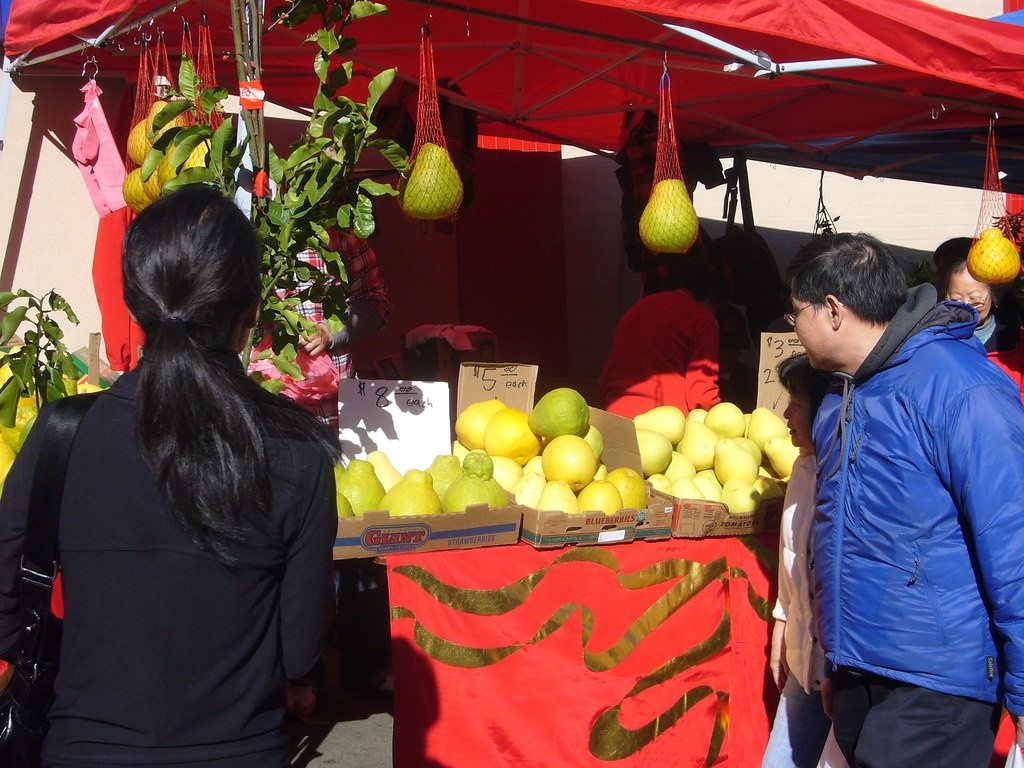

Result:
[266,227,391,434]
[600,255,721,419]
[0,181,339,768]
[762,236,1024,768]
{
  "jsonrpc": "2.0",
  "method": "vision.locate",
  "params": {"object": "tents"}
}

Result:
[0,0,1024,199]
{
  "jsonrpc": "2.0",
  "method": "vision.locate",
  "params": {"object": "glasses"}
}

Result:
[785,299,822,326]
[968,291,991,309]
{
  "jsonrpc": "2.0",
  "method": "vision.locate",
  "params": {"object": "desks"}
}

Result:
[336,532,790,768]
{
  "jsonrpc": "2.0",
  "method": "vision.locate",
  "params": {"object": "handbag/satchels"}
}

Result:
[0,391,99,768]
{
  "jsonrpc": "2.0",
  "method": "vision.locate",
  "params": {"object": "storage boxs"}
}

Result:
[333,489,521,561]
[451,440,674,549]
[644,480,784,537]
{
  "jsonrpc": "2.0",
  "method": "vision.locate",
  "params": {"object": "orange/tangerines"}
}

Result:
[0,345,108,500]
[123,101,212,213]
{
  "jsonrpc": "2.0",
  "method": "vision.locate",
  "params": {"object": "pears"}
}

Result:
[630,402,801,513]
